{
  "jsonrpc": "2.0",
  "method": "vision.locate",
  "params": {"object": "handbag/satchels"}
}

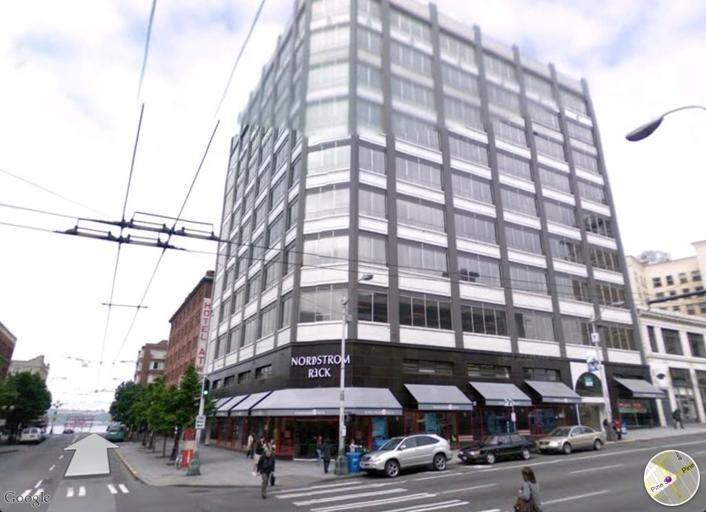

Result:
[271,475,275,486]
[513,497,537,512]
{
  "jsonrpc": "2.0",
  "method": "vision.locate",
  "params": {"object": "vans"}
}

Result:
[18,426,42,444]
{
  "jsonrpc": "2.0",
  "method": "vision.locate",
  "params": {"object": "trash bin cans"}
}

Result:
[180,447,193,467]
[345,452,363,472]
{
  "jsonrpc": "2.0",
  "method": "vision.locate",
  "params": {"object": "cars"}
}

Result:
[105,424,127,442]
[537,424,607,455]
[62,428,74,434]
[455,431,537,465]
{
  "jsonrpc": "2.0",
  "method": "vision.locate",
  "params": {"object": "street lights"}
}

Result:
[332,272,374,474]
[586,299,628,443]
[625,104,705,143]
[47,400,64,435]
[1,405,16,445]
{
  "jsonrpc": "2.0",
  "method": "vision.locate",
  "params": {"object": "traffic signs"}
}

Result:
[194,414,206,431]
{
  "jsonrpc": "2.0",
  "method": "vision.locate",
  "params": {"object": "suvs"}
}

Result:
[359,431,453,479]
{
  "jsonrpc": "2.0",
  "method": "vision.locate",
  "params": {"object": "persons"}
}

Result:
[514,466,543,511]
[602,418,616,440]
[321,437,332,473]
[316,434,322,463]
[244,430,276,474]
[672,408,684,429]
[257,447,275,499]
[349,438,363,452]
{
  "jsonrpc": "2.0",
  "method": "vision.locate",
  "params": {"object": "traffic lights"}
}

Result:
[203,376,210,400]
